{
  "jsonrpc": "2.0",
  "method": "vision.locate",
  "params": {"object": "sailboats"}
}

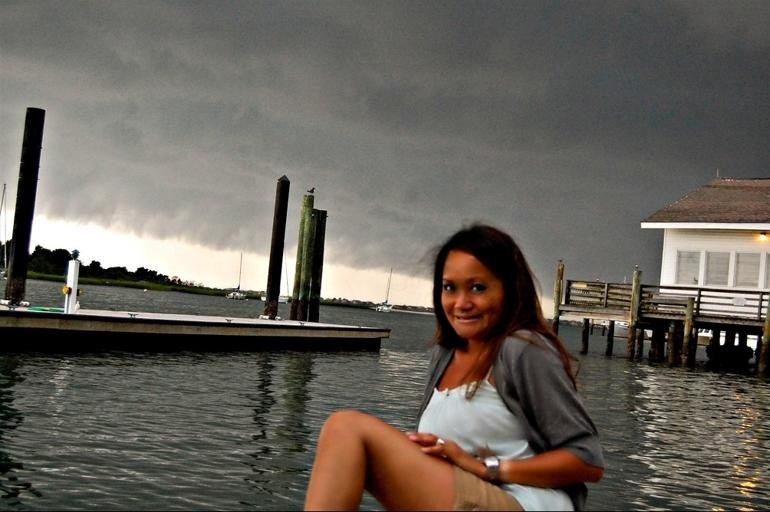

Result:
[223,253,250,303]
[0,183,8,280]
[376,269,395,315]
[261,249,291,304]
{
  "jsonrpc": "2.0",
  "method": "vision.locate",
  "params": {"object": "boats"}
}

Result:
[602,321,714,345]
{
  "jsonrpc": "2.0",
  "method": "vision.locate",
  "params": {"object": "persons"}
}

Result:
[301,224,607,510]
[648,341,665,363]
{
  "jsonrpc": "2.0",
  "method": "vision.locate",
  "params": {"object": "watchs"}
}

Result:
[483,456,501,484]
